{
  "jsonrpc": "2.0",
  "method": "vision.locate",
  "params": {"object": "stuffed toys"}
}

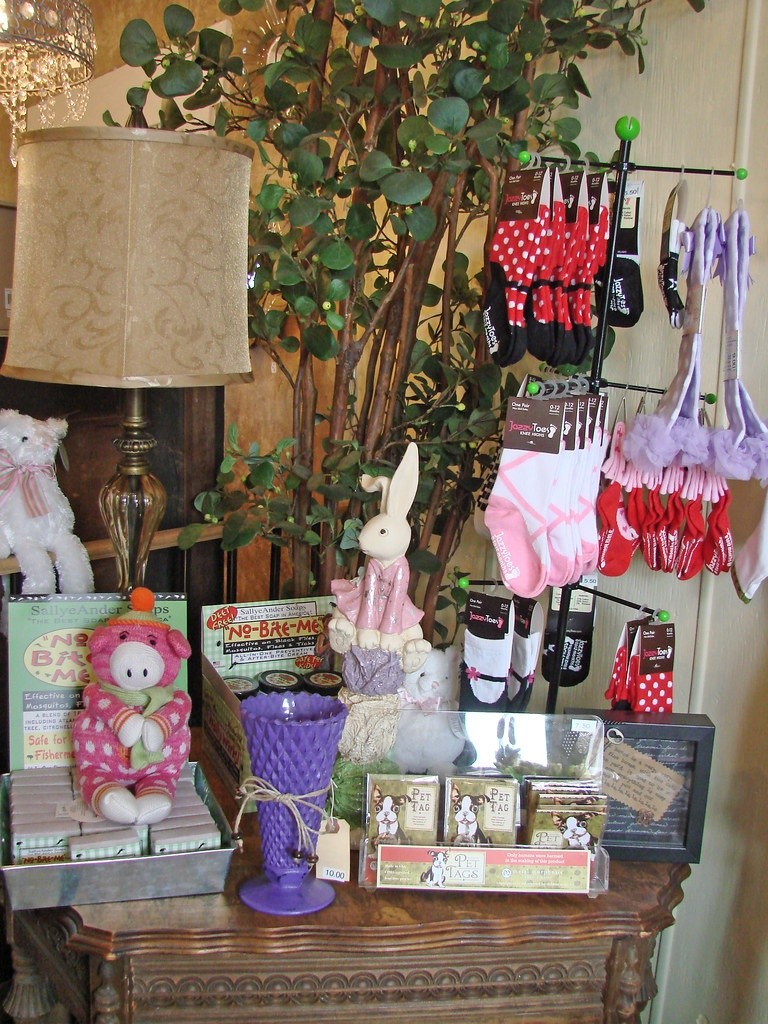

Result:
[71,587,193,827]
[0,409,96,595]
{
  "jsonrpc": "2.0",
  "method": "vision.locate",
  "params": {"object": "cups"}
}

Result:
[240,692,348,916]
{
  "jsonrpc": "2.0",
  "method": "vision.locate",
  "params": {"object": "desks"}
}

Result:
[0,724,692,1024]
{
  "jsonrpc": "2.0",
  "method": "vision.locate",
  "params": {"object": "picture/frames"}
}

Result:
[564,707,715,863]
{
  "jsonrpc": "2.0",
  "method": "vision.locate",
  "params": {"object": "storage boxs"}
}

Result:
[0,761,237,910]
[201,596,348,812]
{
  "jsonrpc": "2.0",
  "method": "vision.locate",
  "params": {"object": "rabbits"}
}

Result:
[329,442,431,672]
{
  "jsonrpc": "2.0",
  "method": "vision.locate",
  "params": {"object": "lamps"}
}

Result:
[1,128,253,592]
[0,0,96,167]
